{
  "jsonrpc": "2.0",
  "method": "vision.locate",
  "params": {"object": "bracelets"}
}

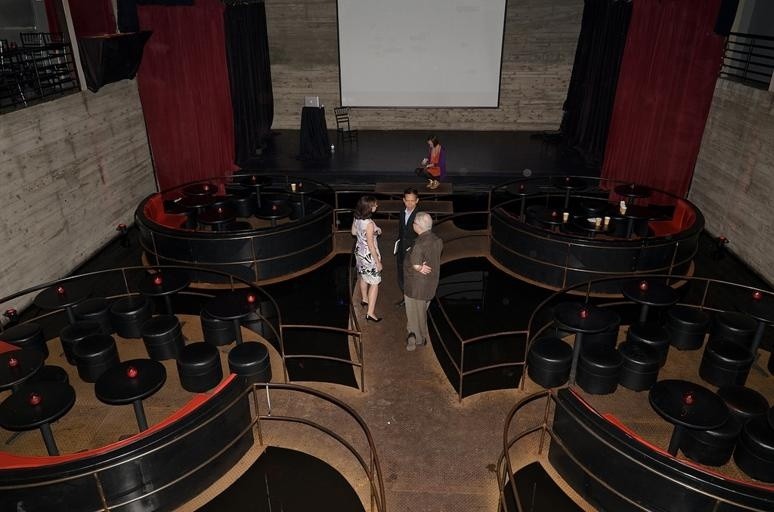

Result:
[418,266,422,272]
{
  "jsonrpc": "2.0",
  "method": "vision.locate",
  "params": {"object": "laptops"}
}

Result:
[304,96,322,107]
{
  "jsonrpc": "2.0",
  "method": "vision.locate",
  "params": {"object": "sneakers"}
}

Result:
[406,332,417,352]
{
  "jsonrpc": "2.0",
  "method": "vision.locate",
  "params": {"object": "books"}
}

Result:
[393,238,400,256]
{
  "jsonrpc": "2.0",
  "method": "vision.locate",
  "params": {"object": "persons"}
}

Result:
[351,195,383,322]
[395,187,424,307]
[402,211,443,351]
[413,134,446,190]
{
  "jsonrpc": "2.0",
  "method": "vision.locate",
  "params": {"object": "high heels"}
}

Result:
[366,314,383,324]
[362,301,369,307]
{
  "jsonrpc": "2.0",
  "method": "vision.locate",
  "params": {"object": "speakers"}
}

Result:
[81,25,154,93]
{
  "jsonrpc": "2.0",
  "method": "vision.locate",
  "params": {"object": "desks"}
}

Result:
[97,360,167,442]
[241,176,271,206]
[614,185,651,204]
[622,279,680,326]
[204,287,263,346]
[33,285,91,325]
[506,183,540,215]
[183,183,217,213]
[735,290,774,358]
[0,380,75,457]
[554,181,587,208]
[646,376,733,459]
[284,184,317,216]
[136,269,196,320]
[548,298,613,387]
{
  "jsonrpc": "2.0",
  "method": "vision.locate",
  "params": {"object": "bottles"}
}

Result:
[330,144,335,153]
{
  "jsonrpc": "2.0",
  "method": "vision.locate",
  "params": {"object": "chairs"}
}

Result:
[0,32,80,107]
[334,106,359,152]
[541,111,572,153]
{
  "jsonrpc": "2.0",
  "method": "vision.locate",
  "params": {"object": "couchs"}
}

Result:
[0,373,253,512]
[134,193,334,284]
[490,198,705,294]
[548,385,774,512]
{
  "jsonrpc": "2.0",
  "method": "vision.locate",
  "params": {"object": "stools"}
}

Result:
[711,307,753,346]
[697,333,757,387]
[575,342,619,396]
[628,317,671,368]
[197,307,232,345]
[666,305,709,352]
[77,297,116,340]
[140,313,182,362]
[615,339,663,394]
[109,298,148,339]
[135,189,304,238]
[529,336,573,388]
[176,341,224,392]
[73,332,122,384]
[733,413,772,485]
[680,410,745,469]
[57,320,103,366]
[531,202,680,245]
[715,380,770,429]
[226,340,274,390]
[581,307,624,345]
[3,323,51,364]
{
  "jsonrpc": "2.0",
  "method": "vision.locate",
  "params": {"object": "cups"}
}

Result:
[604,216,611,226]
[619,200,627,215]
[595,218,602,226]
[563,213,569,222]
